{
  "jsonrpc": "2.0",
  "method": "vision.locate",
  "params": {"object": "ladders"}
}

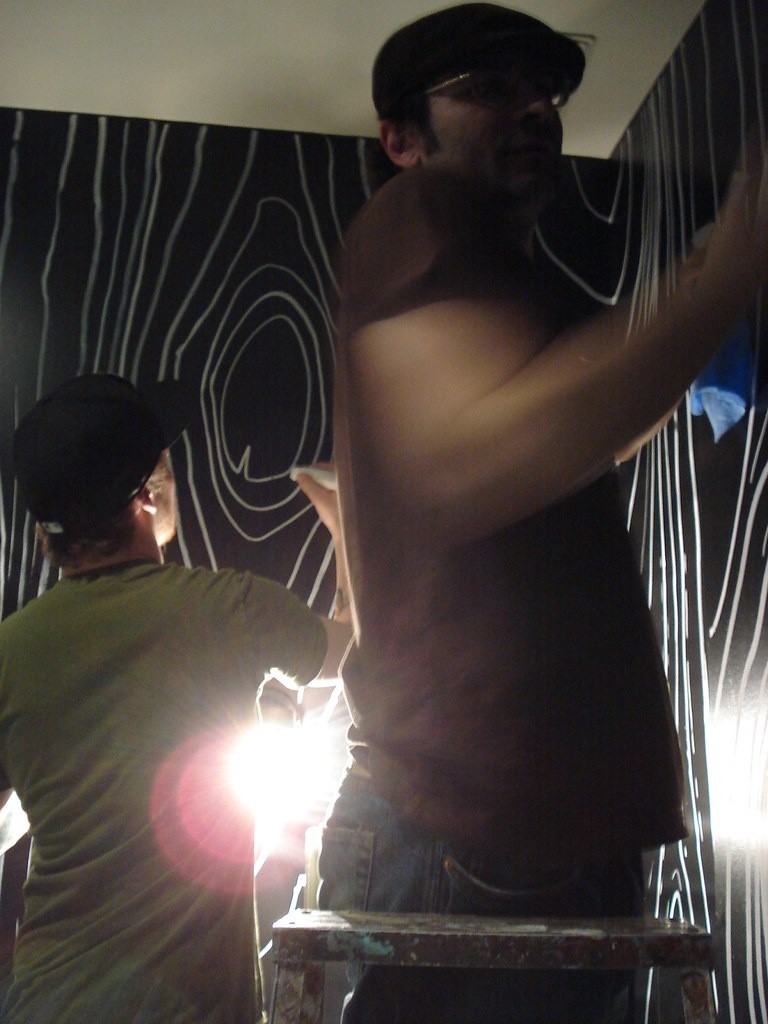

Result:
[268,908,723,1024]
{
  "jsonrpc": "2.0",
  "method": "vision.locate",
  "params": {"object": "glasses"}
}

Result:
[424,47,562,105]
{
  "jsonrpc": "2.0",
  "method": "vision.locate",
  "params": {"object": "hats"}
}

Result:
[373,3,585,116]
[13,371,195,533]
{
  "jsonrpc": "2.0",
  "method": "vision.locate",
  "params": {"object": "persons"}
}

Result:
[0,366,360,1023]
[308,0,768,1024]
